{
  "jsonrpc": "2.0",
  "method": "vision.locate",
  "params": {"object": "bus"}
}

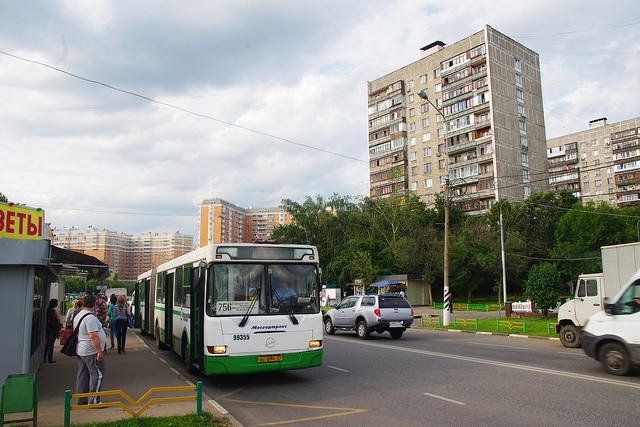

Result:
[134,243,323,375]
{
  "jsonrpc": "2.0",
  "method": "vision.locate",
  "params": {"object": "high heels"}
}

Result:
[110,344,114,349]
[123,348,125,352]
[118,347,122,353]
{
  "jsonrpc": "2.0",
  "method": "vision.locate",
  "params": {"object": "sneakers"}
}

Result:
[48,360,56,363]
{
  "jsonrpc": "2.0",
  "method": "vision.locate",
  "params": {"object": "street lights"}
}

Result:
[417,90,450,326]
[499,197,516,303]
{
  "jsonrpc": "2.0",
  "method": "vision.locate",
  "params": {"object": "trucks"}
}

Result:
[554,242,640,347]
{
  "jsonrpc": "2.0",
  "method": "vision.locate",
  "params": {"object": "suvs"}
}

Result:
[323,294,414,339]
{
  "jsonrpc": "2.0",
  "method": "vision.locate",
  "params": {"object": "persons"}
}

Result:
[400,290,405,297]
[272,279,298,303]
[633,296,640,305]
[74,300,84,317]
[65,298,78,327]
[449,292,453,312]
[72,295,108,408]
[325,294,331,306]
[94,295,108,329]
[108,295,117,349]
[115,295,130,353]
[44,298,64,362]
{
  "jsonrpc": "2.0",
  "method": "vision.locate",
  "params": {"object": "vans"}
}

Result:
[581,270,640,375]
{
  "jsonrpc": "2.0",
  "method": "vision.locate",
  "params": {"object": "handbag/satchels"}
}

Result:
[60,332,79,357]
[50,320,60,332]
[61,328,72,345]
[127,313,134,327]
